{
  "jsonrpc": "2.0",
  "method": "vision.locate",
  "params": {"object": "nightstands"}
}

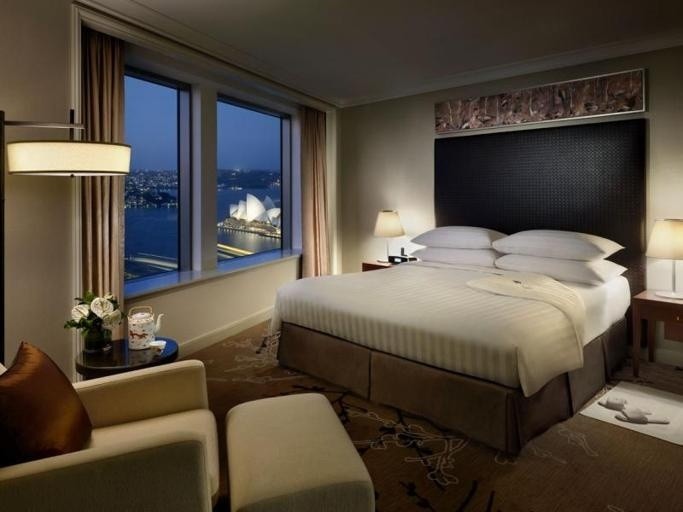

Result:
[362,257,392,272]
[631,291,683,377]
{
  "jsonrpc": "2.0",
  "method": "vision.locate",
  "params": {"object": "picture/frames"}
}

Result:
[435,68,648,135]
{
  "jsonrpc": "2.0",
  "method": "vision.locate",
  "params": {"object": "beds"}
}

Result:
[270,225,633,454]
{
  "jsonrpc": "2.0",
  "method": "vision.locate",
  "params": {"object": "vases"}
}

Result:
[83,327,112,352]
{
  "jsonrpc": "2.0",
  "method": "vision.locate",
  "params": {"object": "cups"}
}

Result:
[148,339,167,355]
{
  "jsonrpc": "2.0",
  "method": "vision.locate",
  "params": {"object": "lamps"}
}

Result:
[373,210,406,263]
[0,109,132,177]
[645,219,683,299]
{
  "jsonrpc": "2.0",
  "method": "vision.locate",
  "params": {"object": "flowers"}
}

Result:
[64,291,122,329]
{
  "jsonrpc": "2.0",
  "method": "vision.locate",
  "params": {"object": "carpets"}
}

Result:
[580,381,683,450]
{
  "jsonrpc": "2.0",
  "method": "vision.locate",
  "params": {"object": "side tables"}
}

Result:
[76,337,178,380]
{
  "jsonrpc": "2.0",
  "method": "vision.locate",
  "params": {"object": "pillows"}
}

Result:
[0,341,92,459]
[411,225,629,285]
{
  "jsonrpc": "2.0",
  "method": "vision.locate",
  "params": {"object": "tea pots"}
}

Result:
[128,349,152,368]
[126,305,164,351]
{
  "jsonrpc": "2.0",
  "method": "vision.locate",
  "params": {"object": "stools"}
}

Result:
[226,392,375,512]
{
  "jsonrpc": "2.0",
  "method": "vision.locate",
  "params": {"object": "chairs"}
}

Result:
[0,360,220,512]
